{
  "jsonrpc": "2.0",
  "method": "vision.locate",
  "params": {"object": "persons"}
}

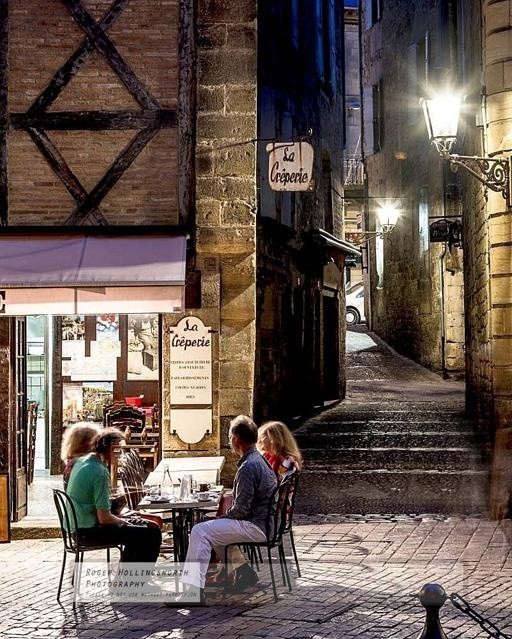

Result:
[164,415,279,606]
[62,421,164,527]
[133,314,154,365]
[63,426,161,562]
[204,420,304,587]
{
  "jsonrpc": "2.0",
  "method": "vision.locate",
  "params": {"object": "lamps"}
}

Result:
[414,70,510,211]
[345,201,403,248]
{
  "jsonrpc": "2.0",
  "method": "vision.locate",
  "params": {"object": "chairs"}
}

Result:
[46,401,303,613]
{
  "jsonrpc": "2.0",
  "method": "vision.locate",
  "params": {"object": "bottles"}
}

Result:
[160,465,173,495]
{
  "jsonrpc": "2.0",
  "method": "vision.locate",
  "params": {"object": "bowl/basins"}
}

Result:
[126,397,144,407]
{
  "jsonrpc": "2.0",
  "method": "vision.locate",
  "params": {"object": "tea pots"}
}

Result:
[199,483,211,492]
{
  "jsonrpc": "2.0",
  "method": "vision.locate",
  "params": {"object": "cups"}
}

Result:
[198,493,209,500]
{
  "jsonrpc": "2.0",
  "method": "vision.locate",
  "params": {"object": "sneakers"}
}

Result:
[233,563,259,592]
[163,593,206,605]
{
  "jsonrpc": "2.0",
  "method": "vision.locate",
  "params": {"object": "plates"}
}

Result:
[151,500,170,504]
[198,499,212,502]
